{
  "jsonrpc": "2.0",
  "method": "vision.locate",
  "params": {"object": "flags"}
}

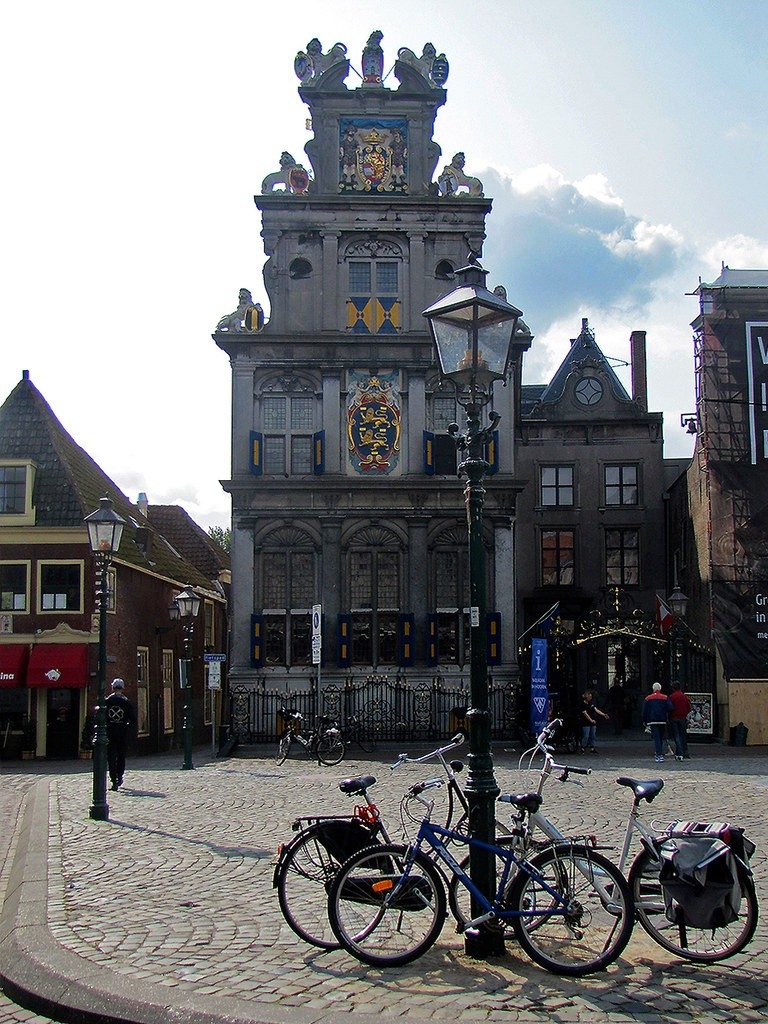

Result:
[656,596,674,636]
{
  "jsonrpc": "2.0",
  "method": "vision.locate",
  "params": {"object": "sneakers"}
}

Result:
[654,754,665,763]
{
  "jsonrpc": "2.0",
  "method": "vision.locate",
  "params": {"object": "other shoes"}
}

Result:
[589,748,600,756]
[108,777,123,790]
[682,753,690,759]
[674,753,683,762]
[578,748,585,755]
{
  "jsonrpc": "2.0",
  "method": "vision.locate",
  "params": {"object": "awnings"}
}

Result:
[26,643,89,688]
[0,643,30,689]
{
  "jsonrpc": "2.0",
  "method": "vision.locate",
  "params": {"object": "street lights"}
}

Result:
[668,585,692,682]
[424,246,524,950]
[173,580,204,771]
[83,490,128,823]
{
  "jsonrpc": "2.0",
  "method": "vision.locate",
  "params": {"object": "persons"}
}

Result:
[669,680,692,759]
[106,678,136,791]
[579,688,609,755]
[608,681,627,736]
[641,682,675,762]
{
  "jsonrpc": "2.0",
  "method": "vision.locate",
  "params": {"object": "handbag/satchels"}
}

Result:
[658,817,756,932]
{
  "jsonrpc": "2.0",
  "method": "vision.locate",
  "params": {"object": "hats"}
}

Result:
[110,678,125,689]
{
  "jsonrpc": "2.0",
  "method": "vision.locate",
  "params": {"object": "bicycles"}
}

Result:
[272,733,537,950]
[329,775,637,977]
[449,717,759,964]
[274,705,377,767]
